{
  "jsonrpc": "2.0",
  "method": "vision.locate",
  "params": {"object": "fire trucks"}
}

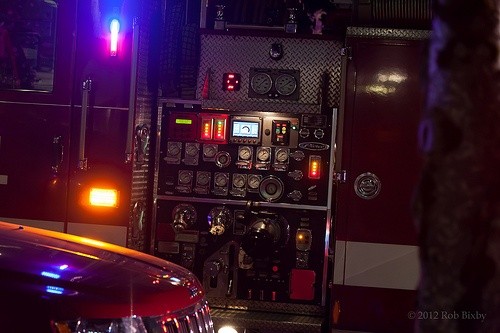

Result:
[0,1,500,332]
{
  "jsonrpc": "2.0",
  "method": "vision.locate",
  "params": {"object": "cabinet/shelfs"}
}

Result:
[145,93,339,321]
